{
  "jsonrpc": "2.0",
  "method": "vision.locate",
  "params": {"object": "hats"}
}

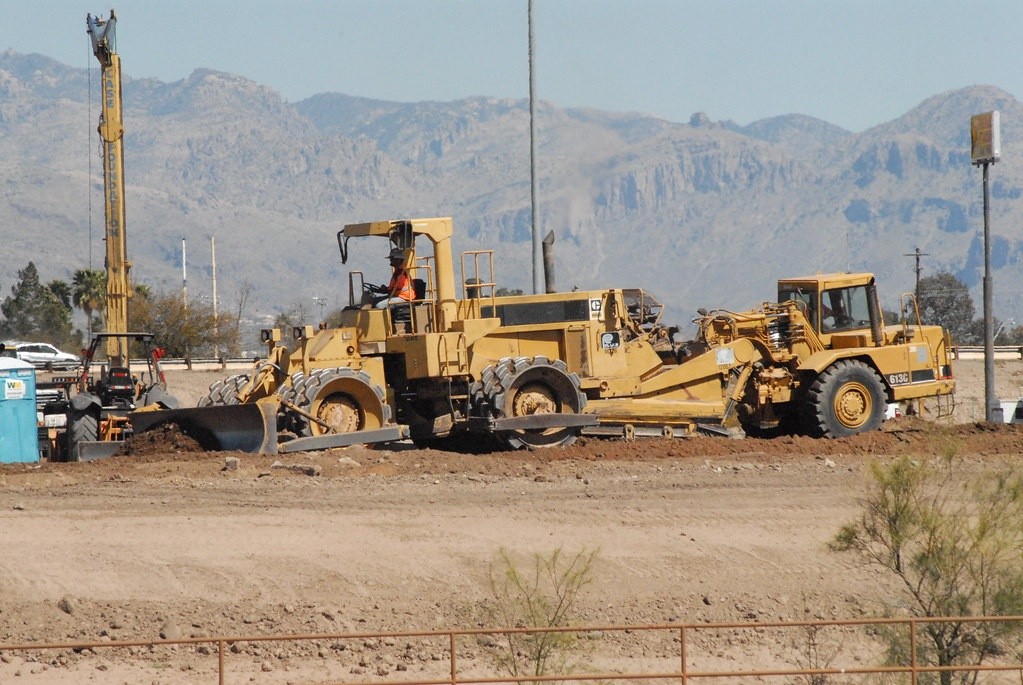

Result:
[385,248,405,259]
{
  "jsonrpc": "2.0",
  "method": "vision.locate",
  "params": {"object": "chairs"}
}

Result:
[391,279,426,323]
[102,368,136,398]
[809,311,832,332]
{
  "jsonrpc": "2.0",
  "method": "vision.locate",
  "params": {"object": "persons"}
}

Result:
[803,295,847,330]
[374,252,416,308]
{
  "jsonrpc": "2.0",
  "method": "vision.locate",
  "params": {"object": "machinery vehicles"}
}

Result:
[125,217,956,451]
[38,8,180,463]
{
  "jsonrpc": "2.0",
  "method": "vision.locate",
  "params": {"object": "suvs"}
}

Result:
[14,342,82,371]
